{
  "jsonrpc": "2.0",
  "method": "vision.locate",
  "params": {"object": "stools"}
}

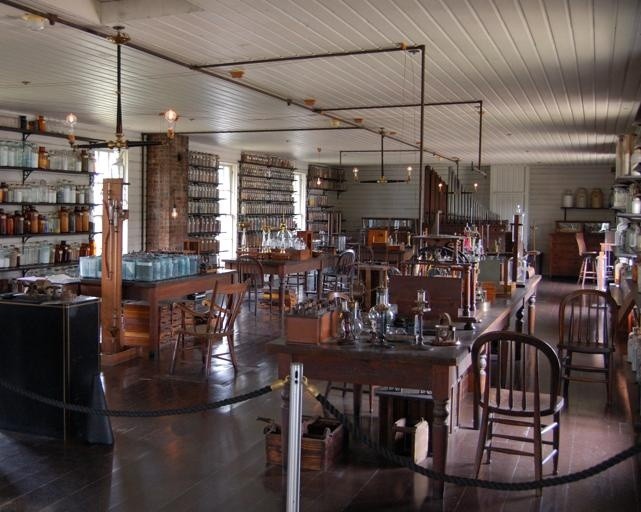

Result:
[577,251,598,289]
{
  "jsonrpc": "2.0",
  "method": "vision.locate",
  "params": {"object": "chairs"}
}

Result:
[558,289,618,407]
[469,331,568,497]
[323,249,357,297]
[167,255,273,375]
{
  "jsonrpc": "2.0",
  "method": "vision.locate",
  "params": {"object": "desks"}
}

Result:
[264,271,546,508]
[81,250,340,363]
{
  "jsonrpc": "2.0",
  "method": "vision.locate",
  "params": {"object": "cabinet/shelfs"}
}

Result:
[549,205,615,277]
[0,126,107,279]
[189,150,220,253]
[308,166,349,233]
[239,151,298,234]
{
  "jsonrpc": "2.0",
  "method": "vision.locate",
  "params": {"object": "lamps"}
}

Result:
[437,159,479,196]
[352,126,415,185]
[66,27,178,149]
[170,188,181,218]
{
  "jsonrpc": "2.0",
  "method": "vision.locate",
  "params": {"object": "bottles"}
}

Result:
[631,197,641,214]
[239,227,306,251]
[630,257,641,294]
[188,151,221,251]
[337,285,426,347]
[458,222,484,262]
[0,140,96,269]
